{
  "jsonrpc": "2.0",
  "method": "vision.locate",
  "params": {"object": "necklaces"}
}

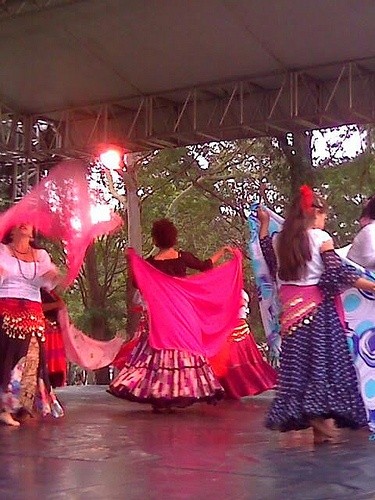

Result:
[10,245,37,281]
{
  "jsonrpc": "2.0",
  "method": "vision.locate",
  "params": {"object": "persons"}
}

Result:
[124,218,234,412]
[257,184,375,445]
[41,272,278,401]
[338,197,375,430]
[0,211,61,427]
